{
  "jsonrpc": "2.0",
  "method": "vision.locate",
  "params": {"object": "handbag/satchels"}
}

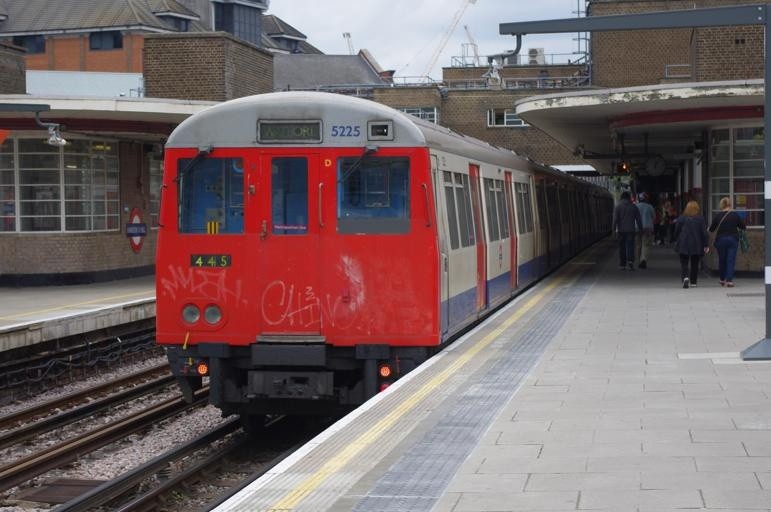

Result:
[706,227,718,247]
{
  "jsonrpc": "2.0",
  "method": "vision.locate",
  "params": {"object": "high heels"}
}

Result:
[718,279,735,287]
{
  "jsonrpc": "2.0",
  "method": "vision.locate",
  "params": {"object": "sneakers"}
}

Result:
[682,277,697,288]
[617,257,647,271]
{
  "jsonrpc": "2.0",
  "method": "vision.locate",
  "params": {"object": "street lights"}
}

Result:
[0,101,68,148]
[482,3,771,361]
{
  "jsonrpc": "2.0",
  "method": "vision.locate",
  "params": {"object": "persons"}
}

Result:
[612,189,746,288]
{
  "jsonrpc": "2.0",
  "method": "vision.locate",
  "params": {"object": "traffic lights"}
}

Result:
[617,160,631,173]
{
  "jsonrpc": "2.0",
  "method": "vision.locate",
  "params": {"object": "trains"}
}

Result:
[156,91,614,436]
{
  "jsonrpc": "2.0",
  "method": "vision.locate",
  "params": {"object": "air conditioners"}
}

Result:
[528,46,545,65]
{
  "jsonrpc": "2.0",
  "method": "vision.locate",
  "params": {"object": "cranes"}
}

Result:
[342,0,480,86]
